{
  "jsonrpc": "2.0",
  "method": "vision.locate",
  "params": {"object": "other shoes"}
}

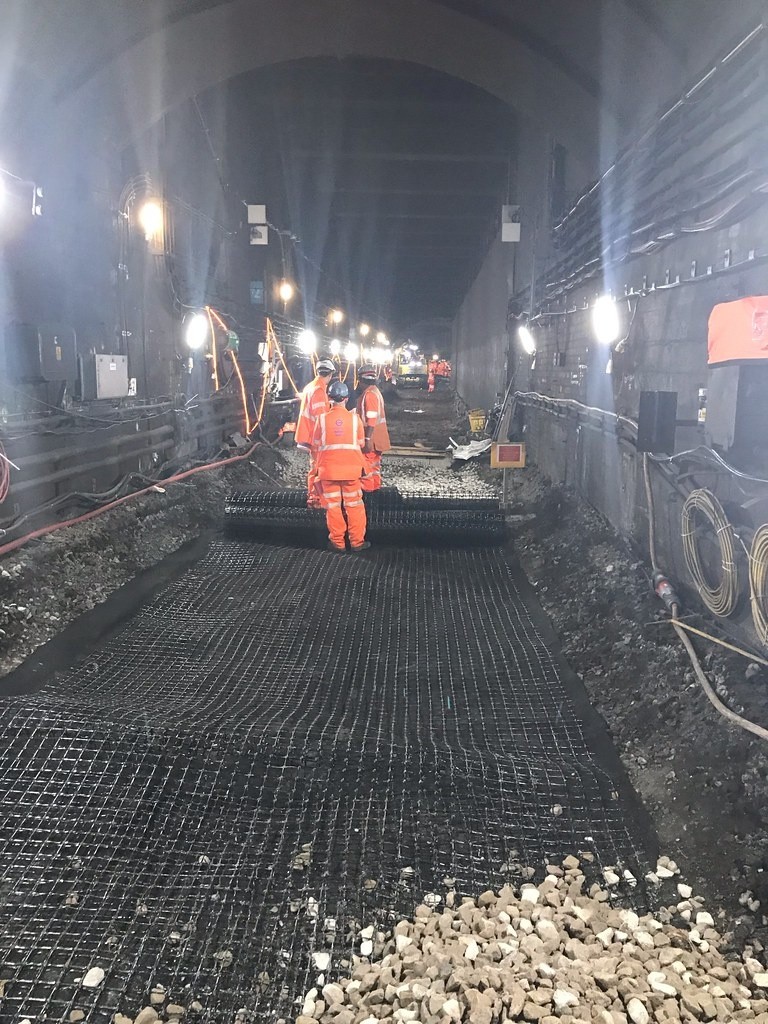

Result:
[353,541,372,552]
[327,541,347,552]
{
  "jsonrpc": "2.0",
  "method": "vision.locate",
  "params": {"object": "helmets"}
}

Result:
[316,357,336,372]
[328,382,348,400]
[357,365,378,380]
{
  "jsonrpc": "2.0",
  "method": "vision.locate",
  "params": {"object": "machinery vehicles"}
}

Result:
[398,354,429,389]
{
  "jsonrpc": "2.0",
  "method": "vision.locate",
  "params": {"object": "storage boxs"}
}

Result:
[469,414,486,432]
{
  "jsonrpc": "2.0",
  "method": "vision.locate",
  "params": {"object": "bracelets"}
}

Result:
[364,437,371,442]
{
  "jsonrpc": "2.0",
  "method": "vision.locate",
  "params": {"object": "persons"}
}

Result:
[426,358,452,392]
[311,381,371,554]
[294,361,391,515]
